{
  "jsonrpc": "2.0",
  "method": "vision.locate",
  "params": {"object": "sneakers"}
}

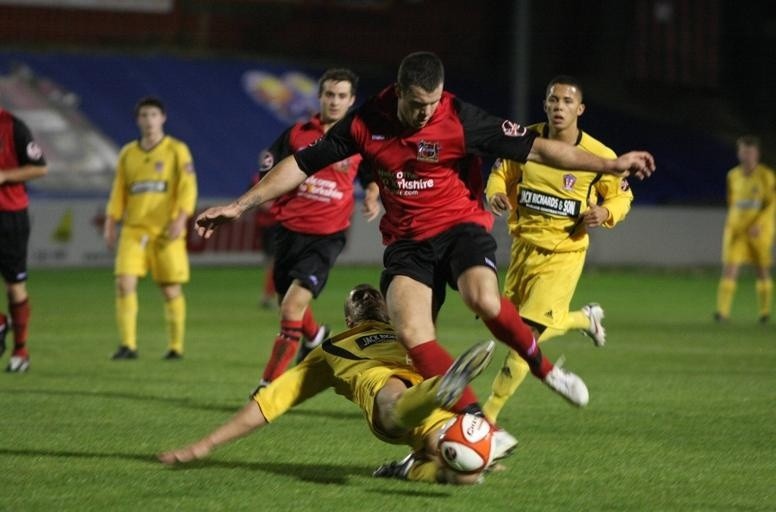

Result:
[373,451,414,478]
[113,346,136,359]
[165,350,182,360]
[543,356,589,408]
[0,314,10,356]
[580,302,606,347]
[435,339,496,408]
[484,428,519,475]
[296,323,332,367]
[6,355,28,372]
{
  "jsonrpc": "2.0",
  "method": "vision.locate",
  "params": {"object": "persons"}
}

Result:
[711,132,776,326]
[103,96,202,361]
[155,281,498,486]
[249,148,274,310]
[247,67,381,406]
[476,75,635,429]
[192,51,658,484]
[0,106,50,373]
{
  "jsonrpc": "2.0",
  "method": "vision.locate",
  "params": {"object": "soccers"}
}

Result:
[437,415,497,473]
